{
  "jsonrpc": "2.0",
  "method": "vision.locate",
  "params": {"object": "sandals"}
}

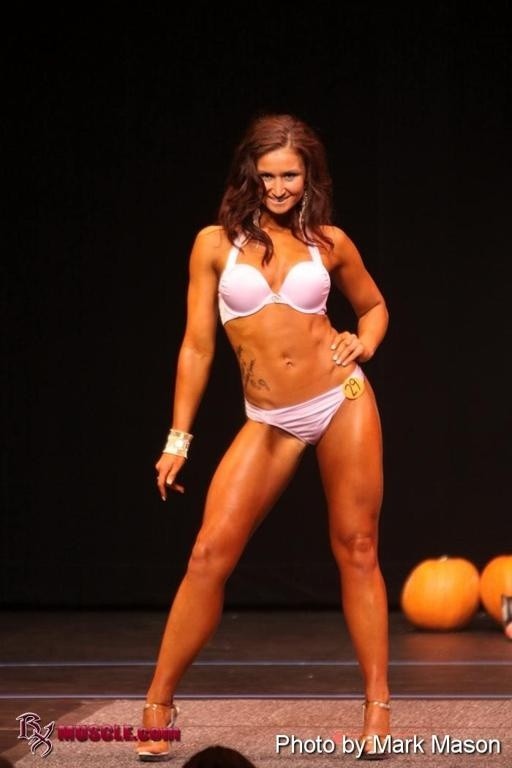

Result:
[360,698,396,761]
[136,701,180,761]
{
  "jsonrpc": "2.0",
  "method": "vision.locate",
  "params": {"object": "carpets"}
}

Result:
[0,692,512,767]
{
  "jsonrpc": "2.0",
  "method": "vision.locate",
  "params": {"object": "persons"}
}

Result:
[130,112,398,762]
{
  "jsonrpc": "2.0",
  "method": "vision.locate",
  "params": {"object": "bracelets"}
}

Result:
[160,424,194,463]
